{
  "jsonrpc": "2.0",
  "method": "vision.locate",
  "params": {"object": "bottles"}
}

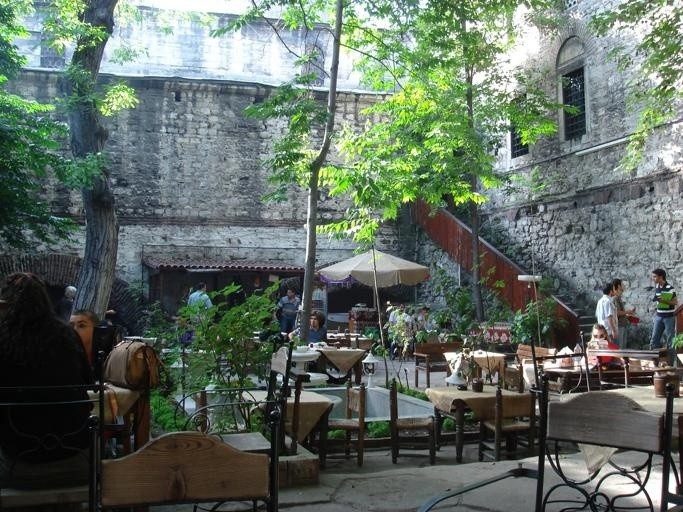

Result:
[573,343,582,360]
[355,335,358,348]
[334,341,340,348]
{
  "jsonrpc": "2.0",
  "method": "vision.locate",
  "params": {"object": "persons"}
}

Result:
[650,269,678,350]
[162,282,212,344]
[587,324,624,370]
[260,282,328,344]
[386,303,439,359]
[596,282,619,345]
[0,269,124,493]
[610,277,636,349]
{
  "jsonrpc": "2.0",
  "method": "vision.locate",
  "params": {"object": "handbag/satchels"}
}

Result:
[102,341,160,389]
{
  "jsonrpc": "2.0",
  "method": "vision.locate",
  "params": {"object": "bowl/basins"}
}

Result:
[296,346,309,353]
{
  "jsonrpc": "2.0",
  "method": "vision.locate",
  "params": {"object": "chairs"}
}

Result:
[0,323,683,512]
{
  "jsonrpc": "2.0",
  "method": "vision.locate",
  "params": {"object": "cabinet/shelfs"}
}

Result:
[349,308,378,332]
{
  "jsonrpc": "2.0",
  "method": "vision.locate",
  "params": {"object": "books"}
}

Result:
[657,291,673,309]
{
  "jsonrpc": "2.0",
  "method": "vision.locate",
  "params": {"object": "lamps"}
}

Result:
[517,274,543,310]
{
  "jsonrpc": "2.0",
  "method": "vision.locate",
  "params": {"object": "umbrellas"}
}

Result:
[318,245,430,346]
